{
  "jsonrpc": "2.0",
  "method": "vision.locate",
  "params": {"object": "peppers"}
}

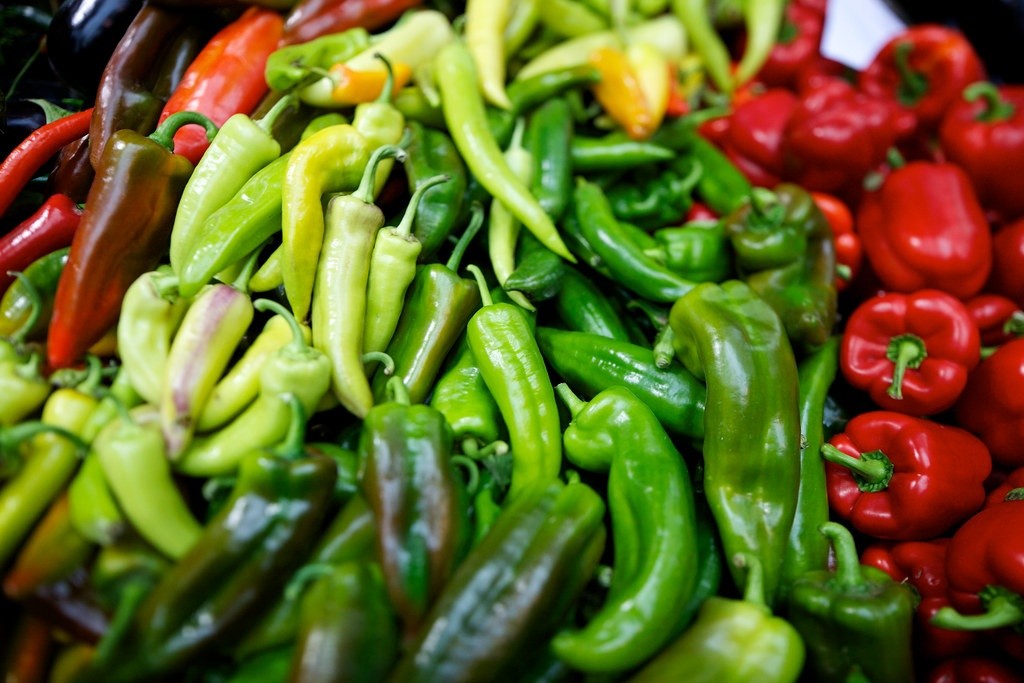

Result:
[0,1,1024,683]
